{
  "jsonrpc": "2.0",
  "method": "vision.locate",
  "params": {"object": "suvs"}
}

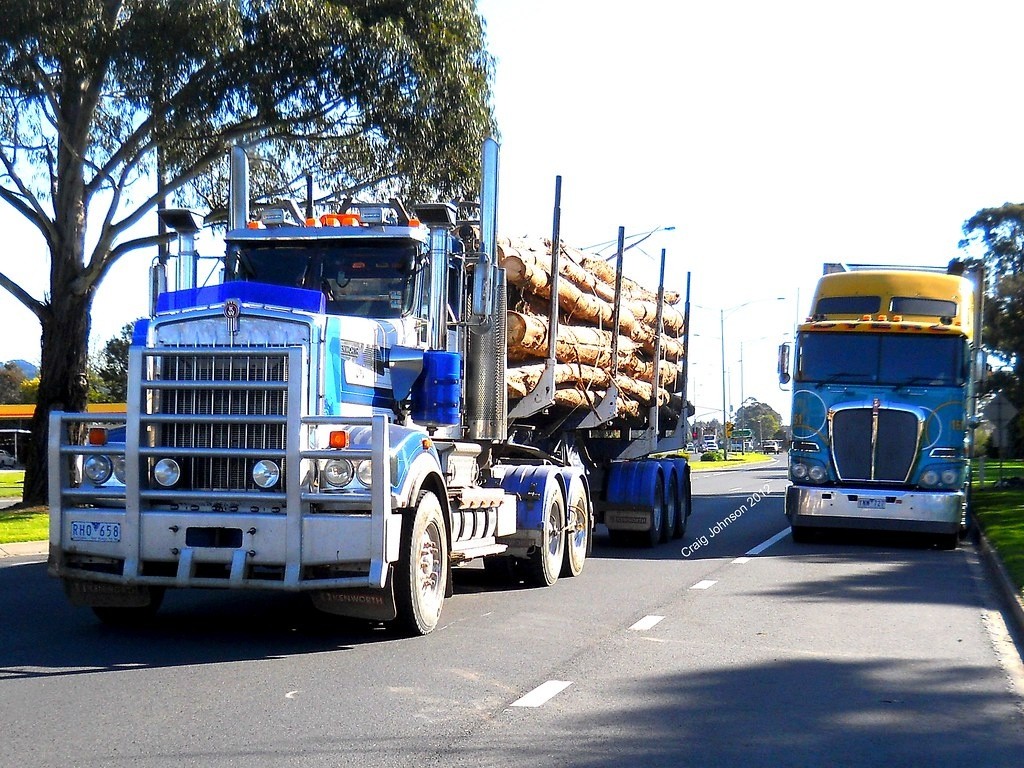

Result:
[763,440,779,456]
[687,443,698,455]
[699,441,720,454]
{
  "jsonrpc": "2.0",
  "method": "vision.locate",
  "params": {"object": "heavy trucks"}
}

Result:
[778,262,990,551]
[49,138,689,636]
[701,426,717,443]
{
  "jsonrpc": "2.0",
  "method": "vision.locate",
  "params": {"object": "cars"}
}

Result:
[778,442,784,453]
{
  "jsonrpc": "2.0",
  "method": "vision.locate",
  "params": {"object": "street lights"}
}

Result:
[720,297,786,462]
[579,228,676,253]
[739,332,788,455]
[689,301,703,365]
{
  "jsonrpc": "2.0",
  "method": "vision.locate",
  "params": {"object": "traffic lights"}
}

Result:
[692,425,699,439]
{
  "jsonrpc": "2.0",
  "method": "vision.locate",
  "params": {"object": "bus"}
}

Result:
[1,403,128,500]
